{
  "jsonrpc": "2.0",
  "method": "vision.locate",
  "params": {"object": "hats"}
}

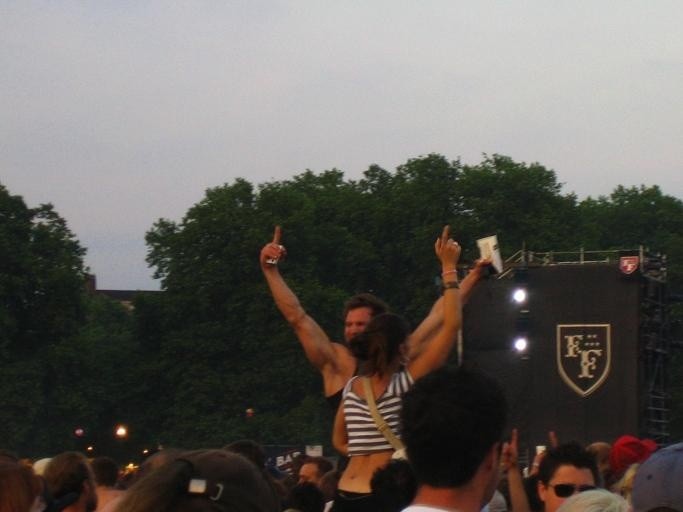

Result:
[632,443,683,512]
[165,448,271,512]
[612,435,658,467]
[33,458,52,475]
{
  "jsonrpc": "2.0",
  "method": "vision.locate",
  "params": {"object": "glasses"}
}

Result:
[547,484,597,498]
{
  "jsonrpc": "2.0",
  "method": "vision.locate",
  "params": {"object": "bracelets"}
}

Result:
[470,269,480,272]
[441,270,458,276]
[440,282,459,296]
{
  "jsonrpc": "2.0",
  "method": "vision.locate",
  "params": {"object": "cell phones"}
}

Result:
[265,244,284,266]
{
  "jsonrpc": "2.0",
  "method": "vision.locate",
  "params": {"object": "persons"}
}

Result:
[401,368,509,512]
[0,439,420,512]
[479,429,682,512]
[332,225,463,512]
[259,225,492,478]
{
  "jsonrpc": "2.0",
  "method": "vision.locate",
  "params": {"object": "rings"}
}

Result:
[453,242,458,246]
